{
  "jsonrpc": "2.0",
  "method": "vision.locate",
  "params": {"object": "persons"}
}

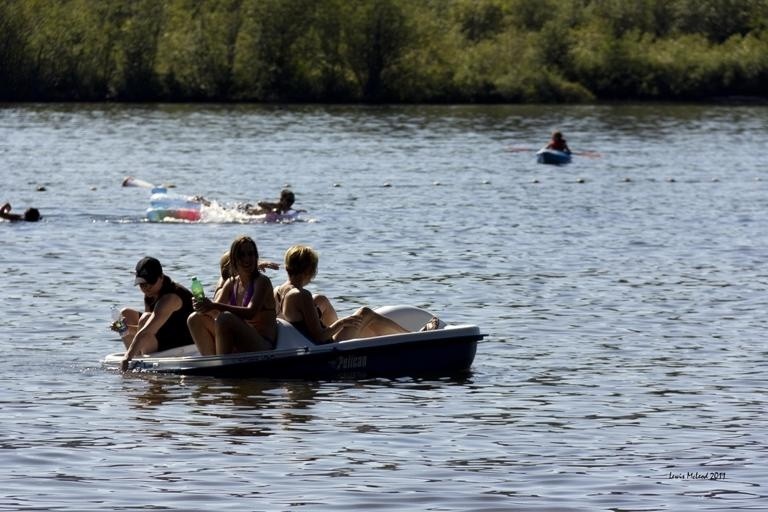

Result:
[186,188,308,217]
[272,246,440,346]
[211,251,338,329]
[119,256,194,373]
[542,131,572,155]
[187,236,279,357]
[0,202,41,222]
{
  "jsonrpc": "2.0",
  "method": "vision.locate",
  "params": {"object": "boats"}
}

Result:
[536,149,571,166]
[146,198,298,224]
[104,304,489,383]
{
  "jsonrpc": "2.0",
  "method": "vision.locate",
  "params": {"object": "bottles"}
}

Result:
[110,305,130,338]
[191,277,207,305]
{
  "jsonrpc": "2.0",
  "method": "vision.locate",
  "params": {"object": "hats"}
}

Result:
[132,256,164,286]
